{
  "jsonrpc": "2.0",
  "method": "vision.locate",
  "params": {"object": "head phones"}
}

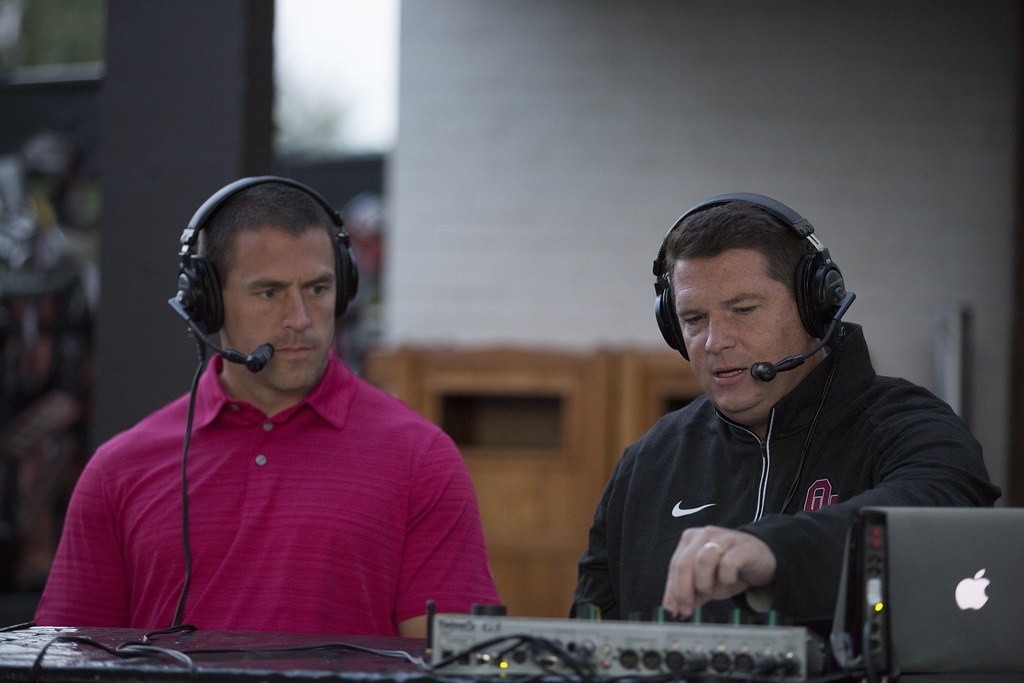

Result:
[653,193,846,362]
[175,175,360,335]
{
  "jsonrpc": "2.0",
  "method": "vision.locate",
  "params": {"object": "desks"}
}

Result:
[0,624,425,683]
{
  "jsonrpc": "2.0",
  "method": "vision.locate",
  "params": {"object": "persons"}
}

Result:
[34,177,508,640]
[568,194,1003,634]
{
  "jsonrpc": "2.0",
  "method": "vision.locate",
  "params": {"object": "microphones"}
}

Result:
[168,298,275,374]
[750,292,857,382]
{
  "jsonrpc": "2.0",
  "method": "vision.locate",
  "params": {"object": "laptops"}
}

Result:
[830,506,1024,683]
